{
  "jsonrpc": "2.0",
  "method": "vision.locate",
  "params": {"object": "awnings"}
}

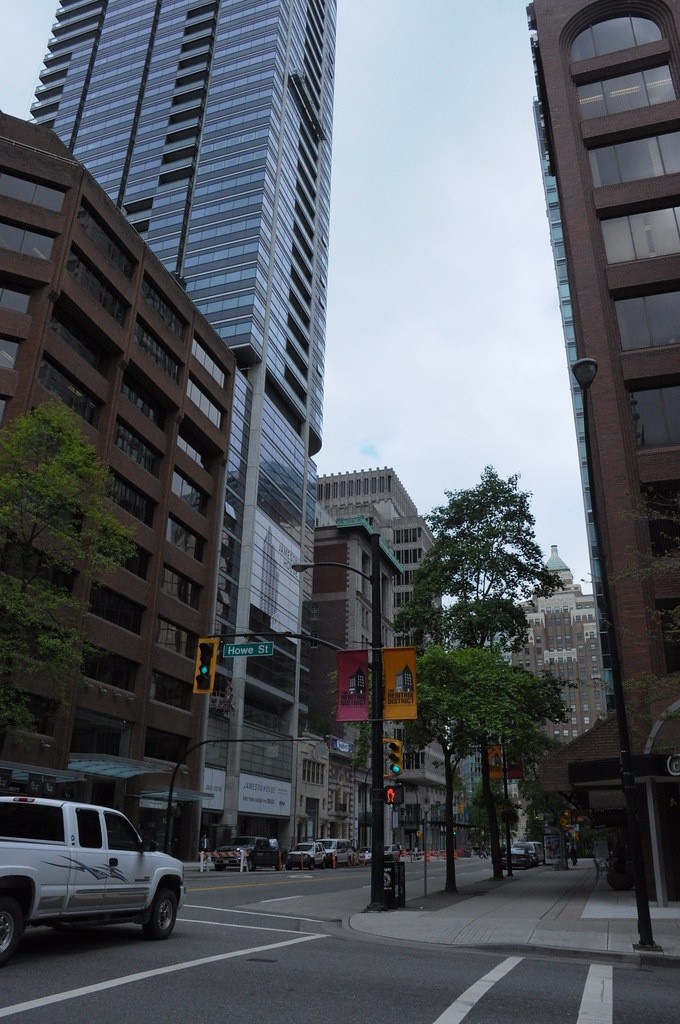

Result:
[0,760,87,782]
[139,786,215,801]
[67,759,174,783]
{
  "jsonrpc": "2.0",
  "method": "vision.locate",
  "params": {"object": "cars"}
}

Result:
[212,836,401,871]
[502,847,533,869]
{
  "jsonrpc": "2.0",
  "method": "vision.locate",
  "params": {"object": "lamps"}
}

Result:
[100,688,107,694]
[42,744,50,748]
[126,697,135,701]
[113,692,122,698]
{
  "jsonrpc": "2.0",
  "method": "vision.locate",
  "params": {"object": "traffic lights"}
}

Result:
[384,785,404,804]
[389,740,403,776]
[193,637,220,694]
[453,828,457,837]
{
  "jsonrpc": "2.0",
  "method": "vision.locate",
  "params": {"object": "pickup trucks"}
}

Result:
[0,797,186,963]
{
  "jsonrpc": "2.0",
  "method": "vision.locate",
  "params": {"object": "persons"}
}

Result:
[481,842,488,859]
[571,845,577,862]
[500,844,506,870]
[201,834,210,864]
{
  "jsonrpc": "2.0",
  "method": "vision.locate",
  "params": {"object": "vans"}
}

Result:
[512,842,544,863]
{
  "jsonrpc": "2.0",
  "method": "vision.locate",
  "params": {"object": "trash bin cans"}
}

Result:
[384,861,405,908]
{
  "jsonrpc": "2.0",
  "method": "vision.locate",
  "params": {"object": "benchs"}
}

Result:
[593,858,610,882]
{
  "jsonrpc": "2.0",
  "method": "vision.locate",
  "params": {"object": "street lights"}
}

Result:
[290,562,384,909]
